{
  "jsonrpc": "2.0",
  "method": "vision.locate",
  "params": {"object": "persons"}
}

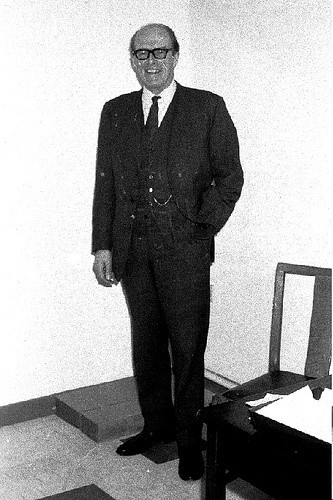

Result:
[90,24,245,481]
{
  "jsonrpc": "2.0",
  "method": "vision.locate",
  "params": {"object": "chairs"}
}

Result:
[211,263,332,405]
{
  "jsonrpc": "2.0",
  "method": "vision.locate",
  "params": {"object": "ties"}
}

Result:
[143,95,161,141]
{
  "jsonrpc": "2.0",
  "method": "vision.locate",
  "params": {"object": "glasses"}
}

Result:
[133,46,176,61]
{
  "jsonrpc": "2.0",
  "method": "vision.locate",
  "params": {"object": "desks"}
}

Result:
[196,374,333,500]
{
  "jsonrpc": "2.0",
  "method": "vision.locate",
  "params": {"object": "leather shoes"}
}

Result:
[176,437,205,481]
[116,424,169,457]
